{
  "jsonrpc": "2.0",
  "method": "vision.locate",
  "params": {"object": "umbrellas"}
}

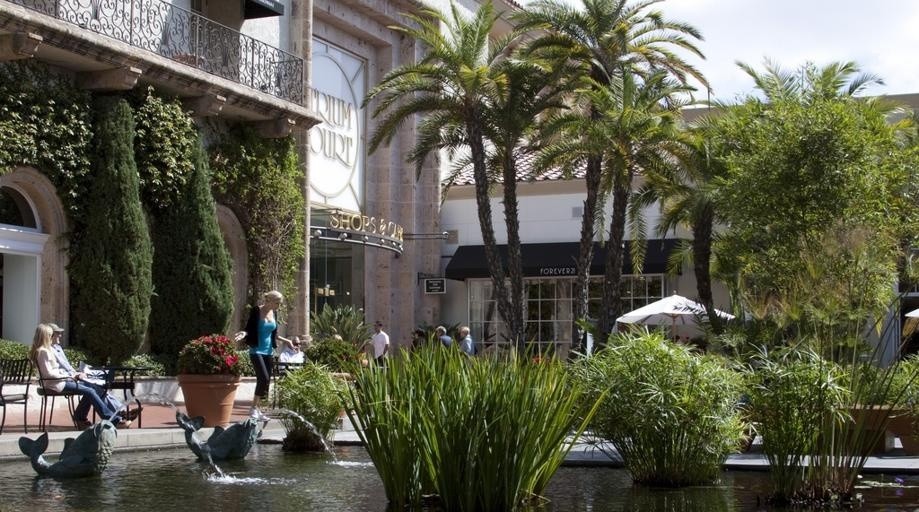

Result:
[612,290,736,344]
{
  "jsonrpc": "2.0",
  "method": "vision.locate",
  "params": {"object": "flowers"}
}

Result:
[176,334,246,377]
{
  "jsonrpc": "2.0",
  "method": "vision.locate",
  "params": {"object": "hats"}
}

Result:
[49,323,63,334]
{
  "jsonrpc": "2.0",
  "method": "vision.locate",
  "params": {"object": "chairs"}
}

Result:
[35,358,80,432]
[0,359,33,435]
[48,395,75,424]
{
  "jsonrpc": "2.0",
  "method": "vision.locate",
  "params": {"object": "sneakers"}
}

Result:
[75,414,91,428]
[249,406,271,422]
[123,408,142,420]
[116,418,131,428]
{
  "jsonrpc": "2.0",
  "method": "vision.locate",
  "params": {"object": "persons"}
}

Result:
[234,290,295,421]
[410,328,427,358]
[460,326,475,357]
[434,326,451,347]
[29,323,132,430]
[369,320,390,366]
[47,323,143,421]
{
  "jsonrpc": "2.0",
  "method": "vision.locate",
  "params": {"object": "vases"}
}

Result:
[176,375,241,427]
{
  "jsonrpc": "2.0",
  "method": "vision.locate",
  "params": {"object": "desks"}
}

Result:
[88,366,154,428]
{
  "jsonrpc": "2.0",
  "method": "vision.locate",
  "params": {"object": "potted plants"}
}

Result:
[710,359,759,452]
[883,352,919,455]
[303,338,363,417]
